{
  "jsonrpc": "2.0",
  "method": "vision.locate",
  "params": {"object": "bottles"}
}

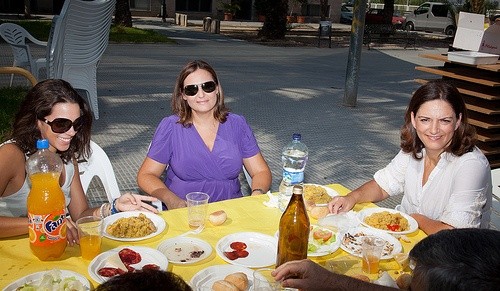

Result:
[277,133,309,213]
[275,184,310,270]
[25,138,68,261]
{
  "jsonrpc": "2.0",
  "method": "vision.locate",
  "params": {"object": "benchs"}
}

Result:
[363,24,418,52]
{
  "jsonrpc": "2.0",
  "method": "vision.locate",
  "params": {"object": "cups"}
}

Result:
[253,267,285,291]
[186,192,211,230]
[361,236,387,274]
[75,216,104,260]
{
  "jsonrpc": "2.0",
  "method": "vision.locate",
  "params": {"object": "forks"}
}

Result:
[182,224,207,236]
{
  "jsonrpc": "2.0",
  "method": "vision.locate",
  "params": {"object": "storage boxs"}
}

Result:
[447,50,499,65]
[451,11,499,55]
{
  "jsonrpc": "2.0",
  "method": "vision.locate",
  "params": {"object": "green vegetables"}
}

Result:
[307,226,336,251]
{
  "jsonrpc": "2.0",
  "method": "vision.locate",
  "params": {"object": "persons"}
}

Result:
[0,78,159,246]
[328,79,493,236]
[137,57,272,211]
[91,268,193,291]
[270,228,500,291]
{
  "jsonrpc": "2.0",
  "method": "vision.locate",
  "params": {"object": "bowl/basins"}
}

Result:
[319,215,350,233]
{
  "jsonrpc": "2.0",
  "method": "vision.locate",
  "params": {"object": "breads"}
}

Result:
[395,273,413,289]
[209,210,226,225]
[304,200,330,219]
[211,280,238,291]
[352,274,370,282]
[394,253,410,266]
[224,272,248,291]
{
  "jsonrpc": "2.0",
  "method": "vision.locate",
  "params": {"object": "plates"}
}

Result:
[98,210,166,242]
[304,184,340,207]
[88,244,168,285]
[3,270,91,291]
[275,224,342,256]
[157,236,214,265]
[216,231,278,268]
[355,208,419,234]
[190,265,271,291]
[336,226,402,259]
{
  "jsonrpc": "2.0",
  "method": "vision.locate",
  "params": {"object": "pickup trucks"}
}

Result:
[489,10,500,21]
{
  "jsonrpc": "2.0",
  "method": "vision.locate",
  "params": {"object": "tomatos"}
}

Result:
[313,231,332,242]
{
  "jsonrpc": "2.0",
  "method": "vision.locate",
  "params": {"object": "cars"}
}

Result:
[406,2,457,35]
[365,8,407,25]
[340,6,353,25]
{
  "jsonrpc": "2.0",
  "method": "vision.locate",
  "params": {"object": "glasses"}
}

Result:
[181,81,217,97]
[36,115,84,133]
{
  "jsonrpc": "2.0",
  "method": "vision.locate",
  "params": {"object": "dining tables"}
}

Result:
[0,184,428,291]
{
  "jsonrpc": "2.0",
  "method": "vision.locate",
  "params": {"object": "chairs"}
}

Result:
[75,136,121,204]
[0,24,49,87]
[44,1,116,120]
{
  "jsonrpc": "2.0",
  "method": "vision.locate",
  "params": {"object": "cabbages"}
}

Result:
[16,271,89,291]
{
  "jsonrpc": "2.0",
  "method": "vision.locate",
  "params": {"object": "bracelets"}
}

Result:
[250,189,265,195]
[100,197,118,219]
